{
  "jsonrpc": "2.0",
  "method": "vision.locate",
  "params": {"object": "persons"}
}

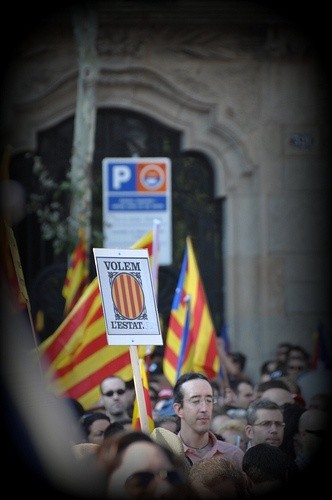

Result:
[61,336,332,500]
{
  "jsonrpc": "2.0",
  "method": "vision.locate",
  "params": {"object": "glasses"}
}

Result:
[102,389,126,397]
[286,364,305,372]
[303,428,332,438]
[252,420,287,429]
[123,470,185,494]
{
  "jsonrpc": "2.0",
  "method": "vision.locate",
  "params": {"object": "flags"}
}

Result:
[31,228,159,410]
[311,323,328,369]
[0,146,28,310]
[131,359,154,435]
[62,213,88,322]
[162,236,221,387]
